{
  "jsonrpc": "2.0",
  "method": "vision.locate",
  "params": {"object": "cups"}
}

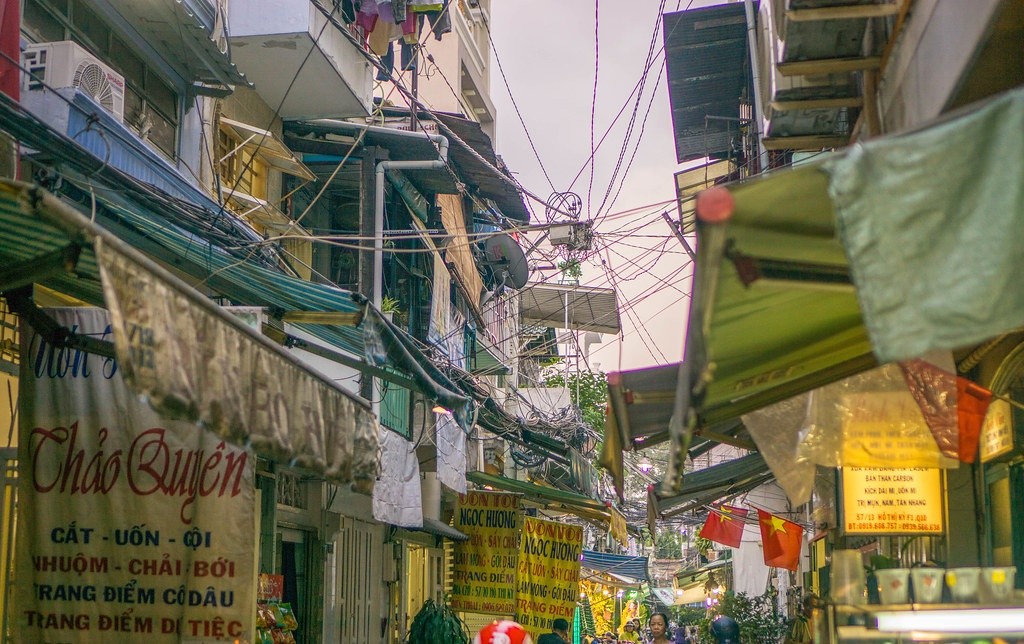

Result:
[874,568,910,604]
[827,549,867,607]
[912,567,944,602]
[981,567,1016,606]
[945,568,981,603]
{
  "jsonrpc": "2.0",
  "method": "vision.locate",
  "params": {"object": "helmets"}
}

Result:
[712,619,739,642]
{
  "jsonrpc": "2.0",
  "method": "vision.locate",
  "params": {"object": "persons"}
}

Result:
[536,619,569,644]
[647,612,676,644]
[605,618,644,644]
[710,616,740,644]
[670,622,697,644]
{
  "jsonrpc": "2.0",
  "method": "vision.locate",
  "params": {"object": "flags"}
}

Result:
[699,504,748,549]
[758,509,803,572]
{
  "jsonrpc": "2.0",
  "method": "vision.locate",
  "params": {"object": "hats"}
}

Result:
[473,620,534,644]
[627,621,634,626]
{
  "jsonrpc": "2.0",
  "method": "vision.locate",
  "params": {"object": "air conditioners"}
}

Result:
[20,40,125,130]
[757,0,873,139]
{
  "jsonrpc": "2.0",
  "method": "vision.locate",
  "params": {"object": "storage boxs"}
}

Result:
[873,566,1017,604]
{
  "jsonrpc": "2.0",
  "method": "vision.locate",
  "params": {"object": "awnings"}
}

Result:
[582,550,651,607]
[521,282,620,336]
[668,90,1024,453]
[469,470,628,550]
[662,0,761,165]
[114,0,255,89]
[0,175,478,497]
[675,558,732,590]
[674,158,737,235]
[436,112,531,222]
[597,362,774,548]
